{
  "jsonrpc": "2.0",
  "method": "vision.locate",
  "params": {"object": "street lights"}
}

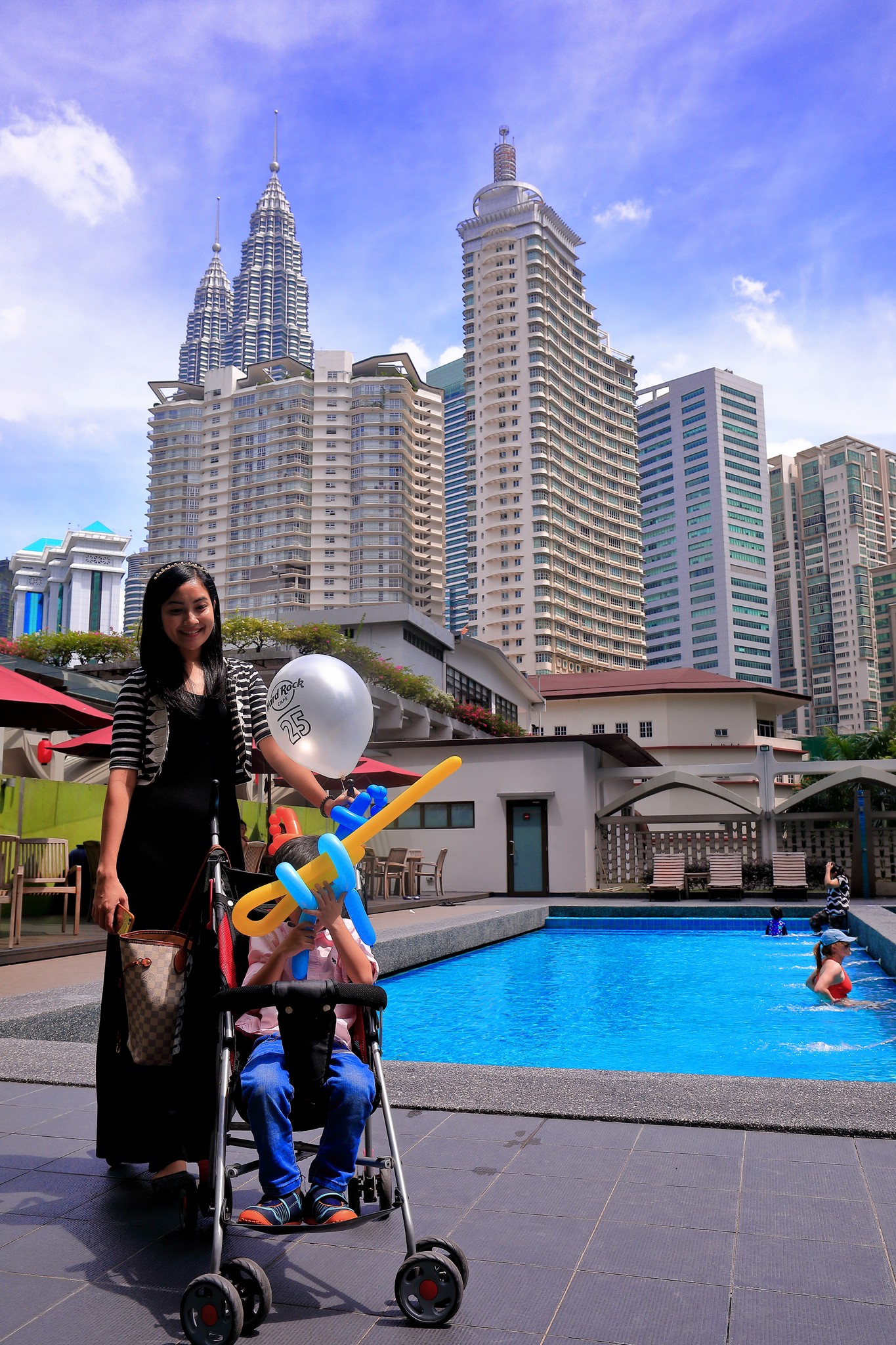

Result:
[271,565,282,651]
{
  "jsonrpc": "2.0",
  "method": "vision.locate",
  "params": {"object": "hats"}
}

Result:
[819,929,857,947]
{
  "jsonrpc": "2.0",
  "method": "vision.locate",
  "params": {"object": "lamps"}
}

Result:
[759,745,770,752]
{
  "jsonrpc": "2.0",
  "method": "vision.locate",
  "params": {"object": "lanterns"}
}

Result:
[37,738,52,765]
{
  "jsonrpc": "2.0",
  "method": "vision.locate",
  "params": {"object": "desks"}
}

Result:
[684,872,711,900]
[359,855,377,900]
[376,855,425,897]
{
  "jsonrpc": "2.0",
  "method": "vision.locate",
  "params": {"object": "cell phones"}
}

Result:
[113,903,135,936]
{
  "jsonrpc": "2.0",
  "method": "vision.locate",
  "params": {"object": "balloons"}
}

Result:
[266,654,374,780]
[232,755,463,981]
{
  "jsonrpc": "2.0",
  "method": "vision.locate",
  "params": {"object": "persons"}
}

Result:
[52,839,100,919]
[229,834,382,1226]
[809,861,851,932]
[806,929,857,1002]
[765,907,788,936]
[90,559,360,1218]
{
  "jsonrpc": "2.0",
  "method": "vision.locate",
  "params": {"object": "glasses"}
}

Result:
[843,943,851,948]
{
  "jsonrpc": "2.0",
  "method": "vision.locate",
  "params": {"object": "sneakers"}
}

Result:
[236,1189,304,1226]
[302,1184,358,1225]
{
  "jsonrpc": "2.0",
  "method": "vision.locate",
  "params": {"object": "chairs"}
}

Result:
[83,840,102,923]
[707,852,744,901]
[772,851,809,902]
[14,837,82,943]
[394,848,424,896]
[367,847,409,900]
[0,834,20,950]
[412,848,448,896]
[243,841,268,874]
[364,847,399,896]
[643,853,688,902]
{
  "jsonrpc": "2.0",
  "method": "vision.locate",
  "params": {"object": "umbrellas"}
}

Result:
[44,726,112,760]
[0,664,114,732]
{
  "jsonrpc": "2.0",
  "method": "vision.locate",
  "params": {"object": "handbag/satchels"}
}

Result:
[118,845,231,1066]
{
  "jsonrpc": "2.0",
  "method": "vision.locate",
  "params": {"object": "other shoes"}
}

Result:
[151,1170,189,1200]
